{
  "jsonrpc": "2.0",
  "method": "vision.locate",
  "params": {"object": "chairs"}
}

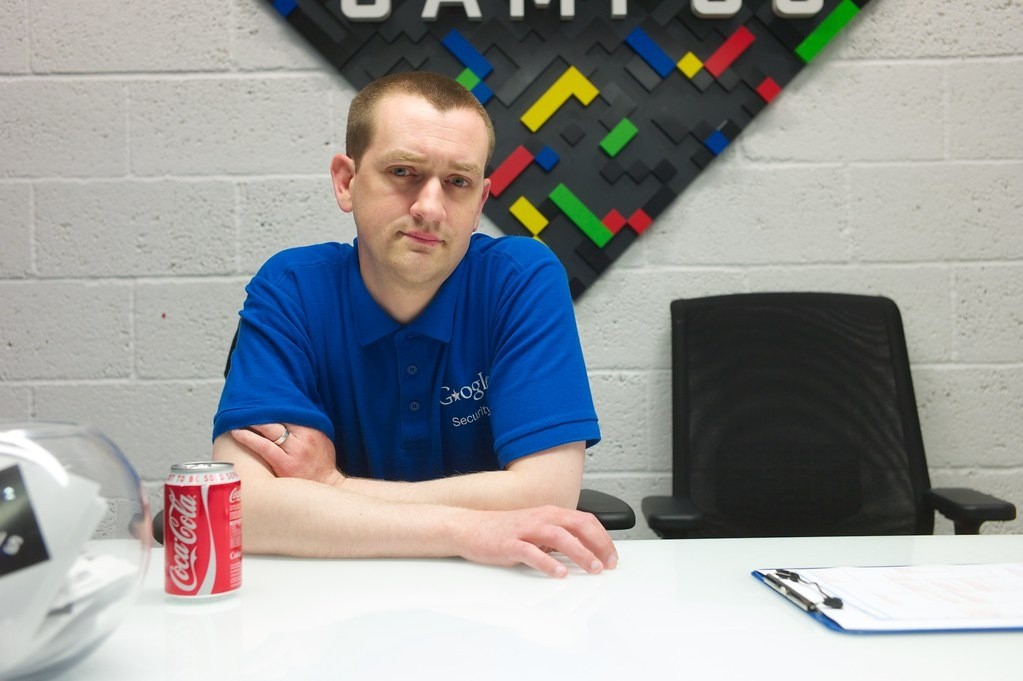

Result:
[641,292,1017,539]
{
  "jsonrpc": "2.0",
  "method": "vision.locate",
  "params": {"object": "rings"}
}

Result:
[275,430,289,445]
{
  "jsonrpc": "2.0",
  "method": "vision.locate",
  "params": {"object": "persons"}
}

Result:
[211,72,620,577]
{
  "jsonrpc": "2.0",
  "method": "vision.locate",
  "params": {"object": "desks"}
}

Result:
[48,533,1023,680]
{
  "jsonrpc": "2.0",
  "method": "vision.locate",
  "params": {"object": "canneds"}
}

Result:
[163,460,244,602]
